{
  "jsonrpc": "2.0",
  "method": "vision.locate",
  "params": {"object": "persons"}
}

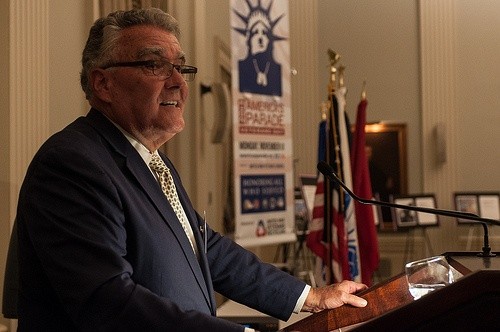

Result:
[3,8,369,332]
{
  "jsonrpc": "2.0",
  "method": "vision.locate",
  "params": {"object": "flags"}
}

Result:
[307,89,379,290]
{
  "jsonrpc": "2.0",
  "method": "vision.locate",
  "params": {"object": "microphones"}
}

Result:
[317,161,500,257]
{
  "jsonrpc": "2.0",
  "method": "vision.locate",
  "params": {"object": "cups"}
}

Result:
[405,255,455,301]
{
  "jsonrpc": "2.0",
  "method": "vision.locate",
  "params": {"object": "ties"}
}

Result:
[148,155,196,254]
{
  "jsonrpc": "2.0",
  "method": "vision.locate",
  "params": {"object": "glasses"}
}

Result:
[100,60,198,81]
